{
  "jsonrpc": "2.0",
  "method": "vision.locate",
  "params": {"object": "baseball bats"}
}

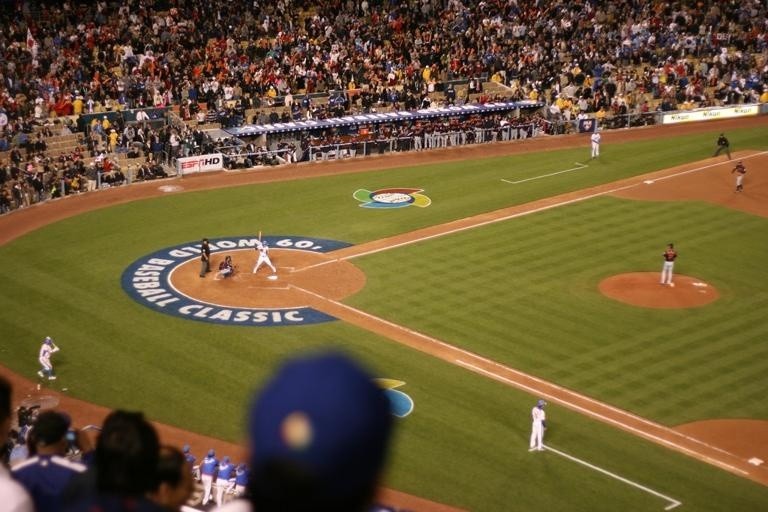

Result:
[259,231,261,242]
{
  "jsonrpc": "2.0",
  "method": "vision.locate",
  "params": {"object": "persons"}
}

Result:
[183,445,247,508]
[0,0,768,204]
[38,337,59,379]
[0,376,193,512]
[245,350,393,512]
[529,399,552,453]
[660,243,679,287]
[189,221,278,281]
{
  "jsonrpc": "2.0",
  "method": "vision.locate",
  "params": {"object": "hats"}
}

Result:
[252,360,388,465]
[34,409,71,445]
[183,445,247,469]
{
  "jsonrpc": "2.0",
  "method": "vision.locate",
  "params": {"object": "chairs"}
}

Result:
[0,76,549,216]
